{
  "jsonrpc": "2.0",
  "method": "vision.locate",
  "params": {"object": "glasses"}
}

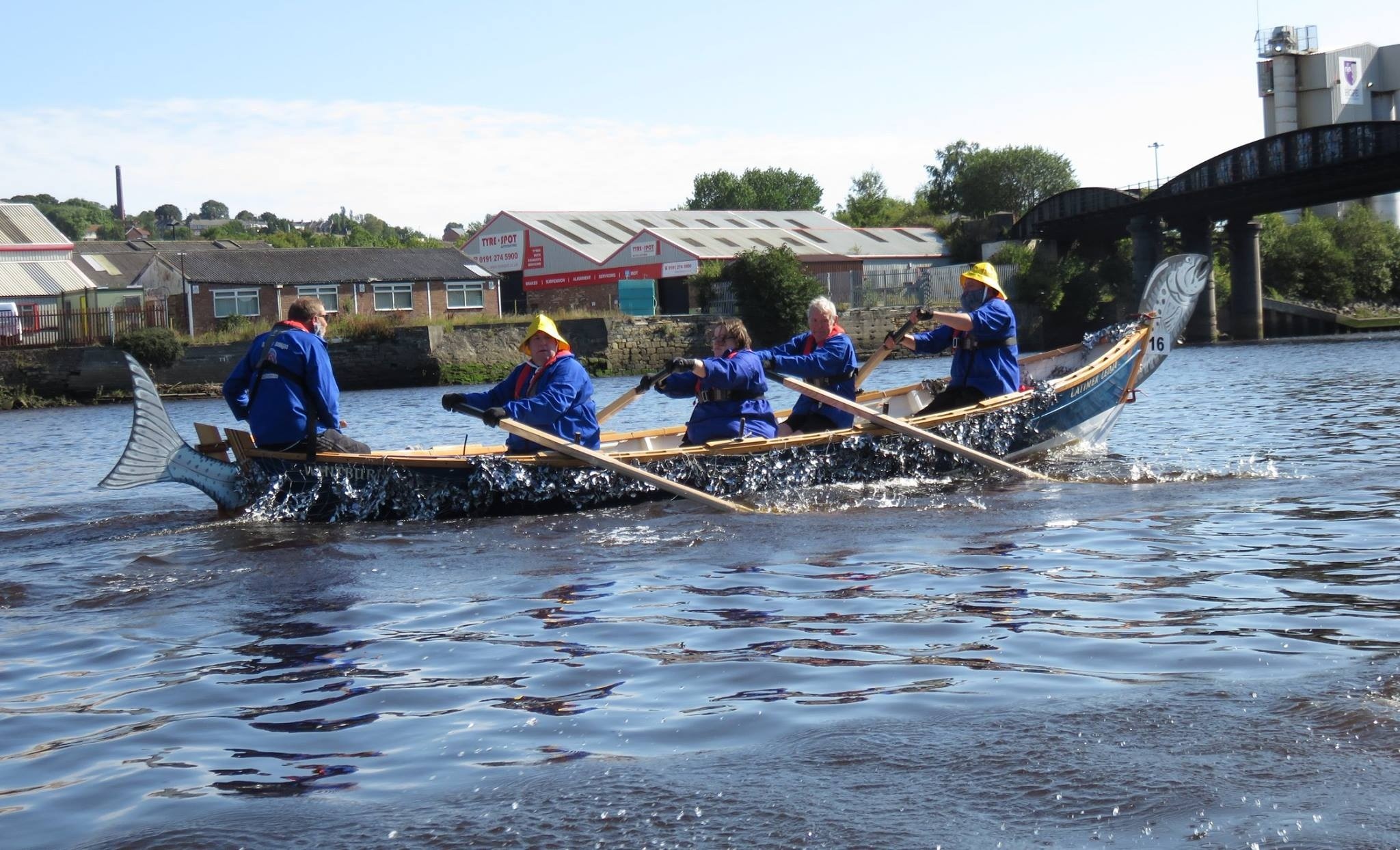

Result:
[310,315,329,322]
[710,334,736,344]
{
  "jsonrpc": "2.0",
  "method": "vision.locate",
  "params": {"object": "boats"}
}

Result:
[89,269,1212,535]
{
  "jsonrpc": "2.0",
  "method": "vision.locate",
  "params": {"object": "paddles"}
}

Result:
[761,369,1054,481]
[855,308,927,390]
[451,398,755,515]
[596,357,684,424]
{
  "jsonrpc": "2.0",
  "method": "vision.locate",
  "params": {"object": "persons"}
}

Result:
[442,315,600,456]
[639,316,777,446]
[884,263,1020,418]
[753,295,859,436]
[223,295,371,455]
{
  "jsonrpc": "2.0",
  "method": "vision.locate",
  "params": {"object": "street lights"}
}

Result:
[176,252,189,332]
[166,222,180,241]
[1148,140,1167,189]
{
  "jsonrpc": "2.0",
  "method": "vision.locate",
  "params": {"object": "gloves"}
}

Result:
[441,393,466,413]
[761,360,774,371]
[662,357,694,374]
[483,407,508,428]
[640,373,667,391]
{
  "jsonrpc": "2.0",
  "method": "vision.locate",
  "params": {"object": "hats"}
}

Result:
[518,314,570,374]
[961,262,1007,309]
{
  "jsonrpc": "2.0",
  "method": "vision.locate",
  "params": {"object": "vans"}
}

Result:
[0,302,23,343]
